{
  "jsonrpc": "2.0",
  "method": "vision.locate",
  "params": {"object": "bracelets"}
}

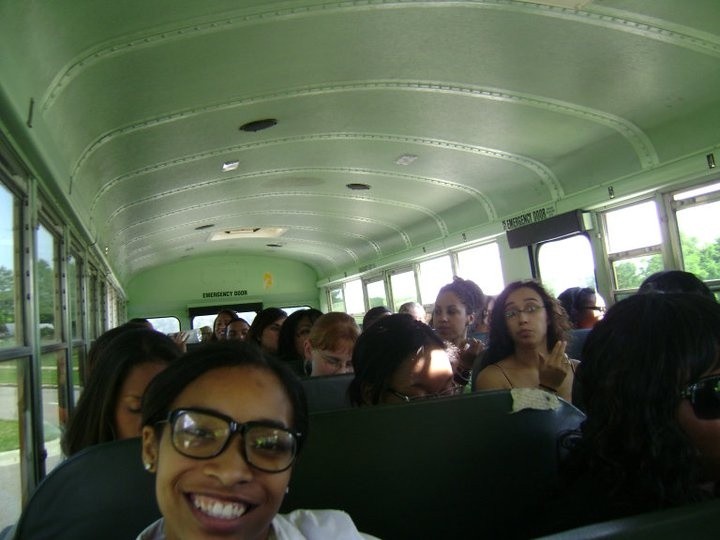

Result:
[456,361,474,374]
[453,368,473,382]
[538,383,557,391]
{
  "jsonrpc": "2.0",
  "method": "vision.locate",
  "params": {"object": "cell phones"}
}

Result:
[183,329,203,344]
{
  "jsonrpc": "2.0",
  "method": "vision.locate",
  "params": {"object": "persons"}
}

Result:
[60,271,720,540]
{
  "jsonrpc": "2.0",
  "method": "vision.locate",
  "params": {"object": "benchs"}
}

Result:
[11,327,720,540]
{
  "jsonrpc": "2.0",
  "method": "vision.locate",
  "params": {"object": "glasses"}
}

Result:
[581,306,606,316]
[392,387,464,402]
[681,375,720,421]
[158,407,301,473]
[502,303,546,318]
